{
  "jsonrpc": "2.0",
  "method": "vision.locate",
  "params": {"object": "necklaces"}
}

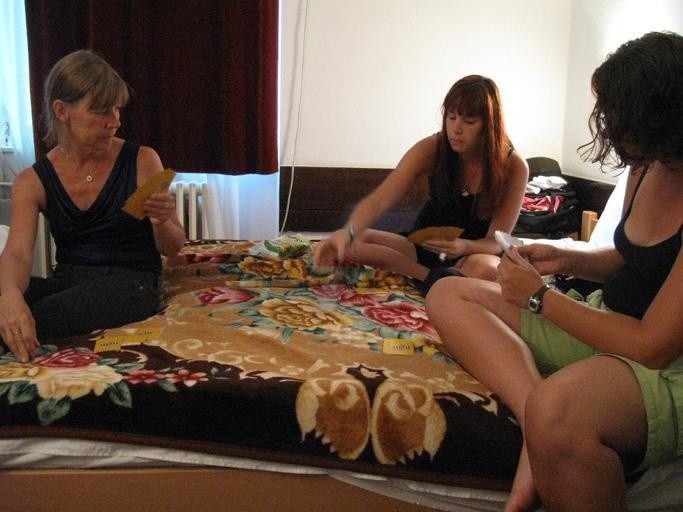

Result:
[459,162,482,197]
[86,173,92,183]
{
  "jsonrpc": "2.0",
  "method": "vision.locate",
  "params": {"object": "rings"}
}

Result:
[438,252,448,263]
[11,330,20,335]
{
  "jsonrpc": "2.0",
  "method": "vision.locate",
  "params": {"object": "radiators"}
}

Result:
[39,182,208,278]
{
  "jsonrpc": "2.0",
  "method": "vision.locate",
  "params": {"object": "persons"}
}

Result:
[0,48,189,364]
[425,31,683,511]
[312,74,530,296]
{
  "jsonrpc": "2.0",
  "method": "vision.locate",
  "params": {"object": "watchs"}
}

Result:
[526,285,550,315]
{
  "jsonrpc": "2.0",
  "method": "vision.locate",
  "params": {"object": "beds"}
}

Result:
[0,163,630,512]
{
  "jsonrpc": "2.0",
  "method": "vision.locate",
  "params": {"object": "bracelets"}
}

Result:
[348,223,356,241]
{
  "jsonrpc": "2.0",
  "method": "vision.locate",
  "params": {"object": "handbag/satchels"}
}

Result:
[515,167,584,237]
